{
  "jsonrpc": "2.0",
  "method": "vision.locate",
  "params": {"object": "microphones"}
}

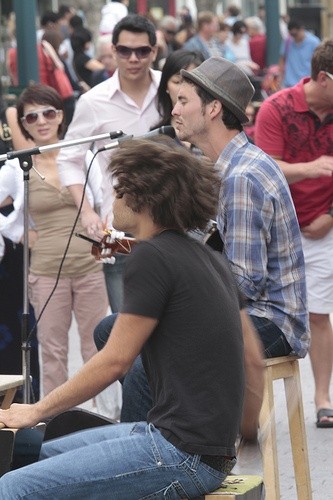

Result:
[99,126,176,152]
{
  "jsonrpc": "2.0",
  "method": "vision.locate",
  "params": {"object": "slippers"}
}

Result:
[316,409,333,427]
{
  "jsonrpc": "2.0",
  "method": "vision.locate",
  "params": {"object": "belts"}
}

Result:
[198,451,236,473]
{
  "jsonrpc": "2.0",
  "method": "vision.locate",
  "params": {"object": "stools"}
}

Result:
[256,354,311,500]
[205,473,264,500]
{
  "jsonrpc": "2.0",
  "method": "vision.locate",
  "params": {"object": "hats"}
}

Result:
[180,57,254,124]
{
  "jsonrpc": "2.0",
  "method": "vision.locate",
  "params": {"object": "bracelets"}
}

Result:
[239,435,258,446]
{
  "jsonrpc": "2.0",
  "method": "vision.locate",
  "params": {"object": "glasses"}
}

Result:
[115,46,154,59]
[22,109,60,124]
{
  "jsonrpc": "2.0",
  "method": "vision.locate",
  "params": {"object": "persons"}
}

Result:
[0,134,265,500]
[55,14,163,313]
[0,83,121,421]
[148,48,211,154]
[253,38,333,428]
[94,56,310,385]
[6,0,320,127]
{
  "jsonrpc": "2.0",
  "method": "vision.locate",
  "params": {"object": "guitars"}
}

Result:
[72,218,223,264]
